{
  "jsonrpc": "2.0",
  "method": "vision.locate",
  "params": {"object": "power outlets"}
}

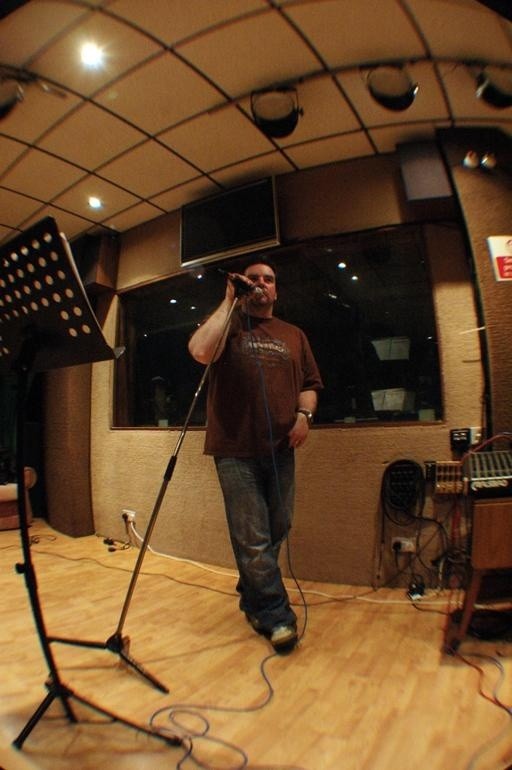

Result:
[120,509,136,524]
[392,537,415,552]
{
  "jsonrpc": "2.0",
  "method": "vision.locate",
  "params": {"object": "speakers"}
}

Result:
[78,233,120,292]
[395,140,453,203]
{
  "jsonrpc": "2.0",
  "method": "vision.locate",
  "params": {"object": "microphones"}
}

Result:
[218,267,264,299]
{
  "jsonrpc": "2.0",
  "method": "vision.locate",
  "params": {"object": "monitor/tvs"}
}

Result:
[181,176,280,266]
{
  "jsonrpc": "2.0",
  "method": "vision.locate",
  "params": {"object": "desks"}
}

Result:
[444,499,512,657]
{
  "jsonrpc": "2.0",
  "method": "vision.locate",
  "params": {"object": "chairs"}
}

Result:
[0,466,37,532]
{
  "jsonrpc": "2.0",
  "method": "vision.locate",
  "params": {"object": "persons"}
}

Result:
[188,253,325,646]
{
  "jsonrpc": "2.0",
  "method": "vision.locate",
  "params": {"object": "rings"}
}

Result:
[295,439,300,446]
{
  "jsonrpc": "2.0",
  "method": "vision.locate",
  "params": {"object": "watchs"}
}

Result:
[296,408,315,428]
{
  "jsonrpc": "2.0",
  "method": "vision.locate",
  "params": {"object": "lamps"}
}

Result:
[2,63,36,125]
[244,56,512,138]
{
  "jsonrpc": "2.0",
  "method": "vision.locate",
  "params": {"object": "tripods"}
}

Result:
[50,314,172,696]
[0,479,182,770]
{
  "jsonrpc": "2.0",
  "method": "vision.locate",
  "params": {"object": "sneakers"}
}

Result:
[271,625,298,647]
